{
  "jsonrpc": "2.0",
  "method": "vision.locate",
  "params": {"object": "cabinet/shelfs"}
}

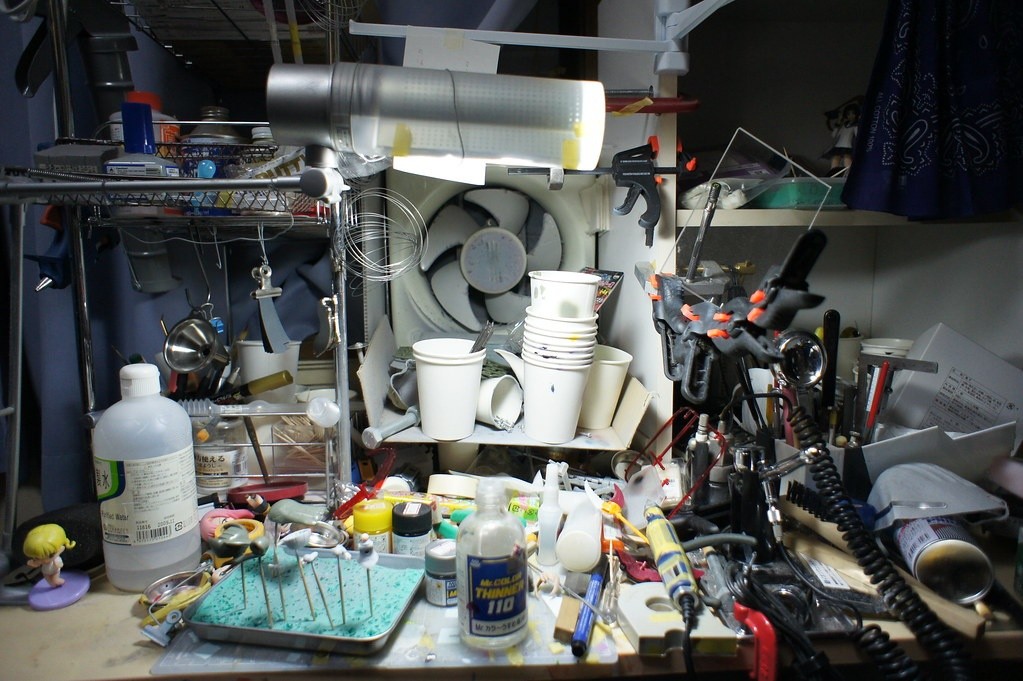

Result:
[49,0,359,497]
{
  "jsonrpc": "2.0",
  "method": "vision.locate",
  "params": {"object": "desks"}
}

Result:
[0,544,1023,681]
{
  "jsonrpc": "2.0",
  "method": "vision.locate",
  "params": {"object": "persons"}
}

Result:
[818,106,861,175]
[23,523,76,588]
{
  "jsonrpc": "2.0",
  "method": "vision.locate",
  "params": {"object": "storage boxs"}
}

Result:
[775,321,1023,502]
[761,177,848,209]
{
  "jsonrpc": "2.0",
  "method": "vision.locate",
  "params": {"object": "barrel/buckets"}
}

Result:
[837,334,915,387]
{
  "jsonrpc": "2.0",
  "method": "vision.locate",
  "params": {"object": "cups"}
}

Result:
[412,270,633,445]
[236,339,302,406]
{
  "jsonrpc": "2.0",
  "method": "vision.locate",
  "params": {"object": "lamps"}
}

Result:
[266,0,734,190]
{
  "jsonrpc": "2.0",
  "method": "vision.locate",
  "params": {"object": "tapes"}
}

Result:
[214,519,264,570]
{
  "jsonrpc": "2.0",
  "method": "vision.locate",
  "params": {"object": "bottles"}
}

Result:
[555,500,603,573]
[105,92,289,212]
[455,483,528,650]
[424,538,458,606]
[893,516,995,604]
[353,500,432,558]
[537,465,560,567]
[92,363,202,592]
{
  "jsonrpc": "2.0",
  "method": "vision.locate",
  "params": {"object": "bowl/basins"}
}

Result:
[751,177,847,210]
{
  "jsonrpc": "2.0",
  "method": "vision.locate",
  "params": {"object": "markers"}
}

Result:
[570,573,603,656]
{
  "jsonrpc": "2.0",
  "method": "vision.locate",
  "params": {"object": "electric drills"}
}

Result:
[26,198,119,292]
